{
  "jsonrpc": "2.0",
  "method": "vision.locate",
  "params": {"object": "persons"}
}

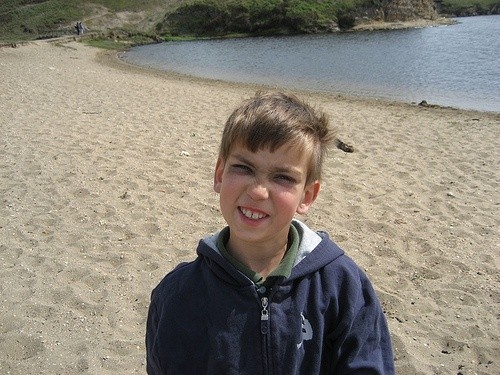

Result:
[144,93,394,375]
[76,21,84,35]
